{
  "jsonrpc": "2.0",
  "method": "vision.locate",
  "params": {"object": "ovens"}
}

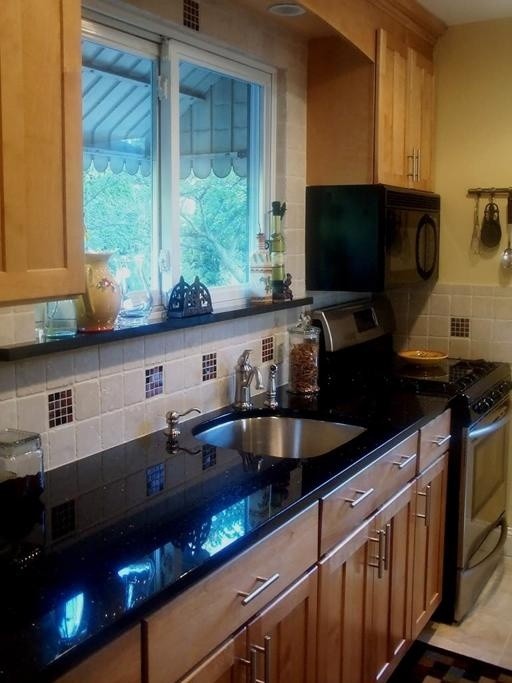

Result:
[454,392,512,624]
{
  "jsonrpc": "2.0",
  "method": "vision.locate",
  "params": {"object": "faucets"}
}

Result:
[166,436,202,456]
[241,453,263,475]
[234,349,264,409]
[165,406,201,435]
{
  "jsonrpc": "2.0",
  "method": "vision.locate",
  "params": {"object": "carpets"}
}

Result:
[385,638,512,683]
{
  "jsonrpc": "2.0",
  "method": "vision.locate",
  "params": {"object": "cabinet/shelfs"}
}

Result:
[414,452,453,646]
[319,480,416,681]
[319,427,420,562]
[306,1,450,194]
[146,497,321,683]
[419,415,450,472]
[1,0,86,302]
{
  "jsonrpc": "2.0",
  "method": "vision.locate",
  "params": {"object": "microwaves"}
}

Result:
[306,184,440,294]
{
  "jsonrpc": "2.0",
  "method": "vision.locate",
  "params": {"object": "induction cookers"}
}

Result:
[334,354,512,428]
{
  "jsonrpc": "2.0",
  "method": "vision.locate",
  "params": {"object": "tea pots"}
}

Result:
[73,249,154,332]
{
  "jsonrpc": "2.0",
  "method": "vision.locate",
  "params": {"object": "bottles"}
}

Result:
[1,427,46,504]
[250,201,286,305]
[287,314,322,395]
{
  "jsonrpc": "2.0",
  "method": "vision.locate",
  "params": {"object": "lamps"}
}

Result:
[271,4,304,17]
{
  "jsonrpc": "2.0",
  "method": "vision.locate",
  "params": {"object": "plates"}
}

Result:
[398,349,447,367]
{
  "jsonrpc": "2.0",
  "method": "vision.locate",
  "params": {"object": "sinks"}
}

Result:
[195,417,368,458]
